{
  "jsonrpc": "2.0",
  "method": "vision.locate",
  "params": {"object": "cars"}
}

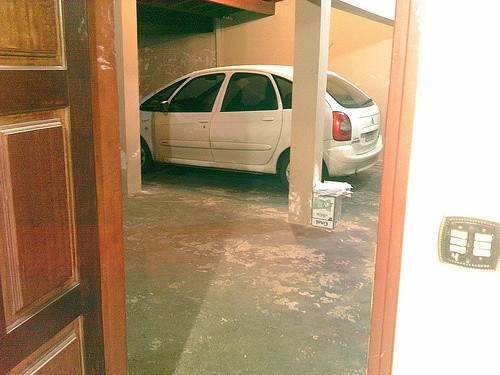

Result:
[138,64,384,193]
